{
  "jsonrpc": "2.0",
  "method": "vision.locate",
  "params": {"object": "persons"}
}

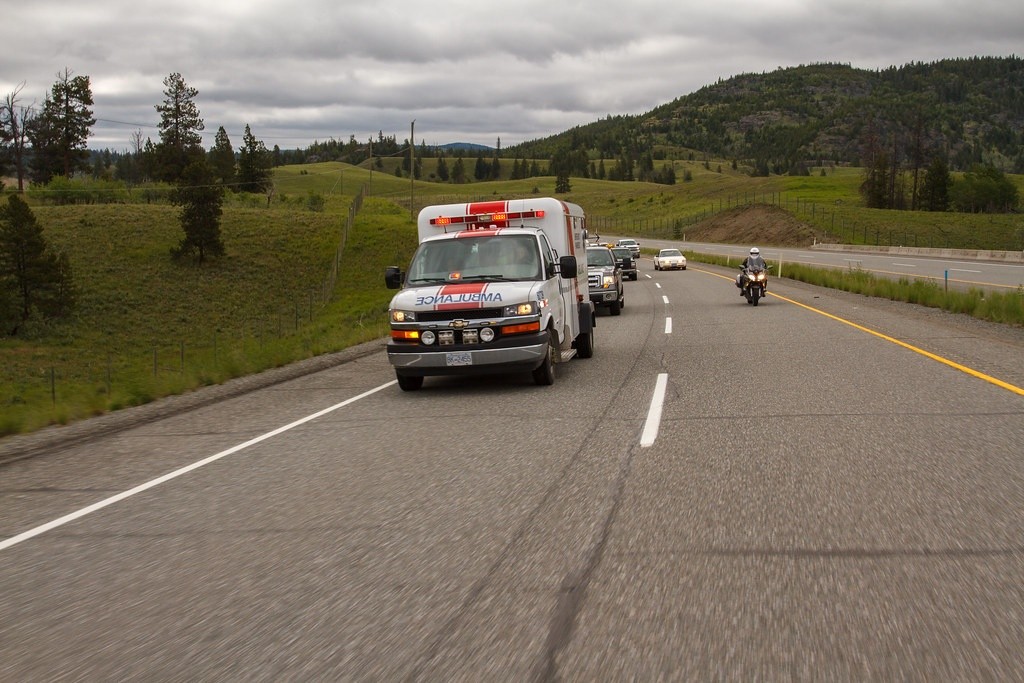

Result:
[740,248,768,297]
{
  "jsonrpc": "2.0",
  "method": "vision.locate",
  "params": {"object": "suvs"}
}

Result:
[616,239,640,258]
[587,246,625,316]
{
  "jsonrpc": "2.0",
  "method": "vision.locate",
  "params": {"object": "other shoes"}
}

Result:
[740,291,746,296]
[761,292,765,297]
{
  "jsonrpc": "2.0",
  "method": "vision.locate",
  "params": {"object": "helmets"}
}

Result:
[750,247,759,259]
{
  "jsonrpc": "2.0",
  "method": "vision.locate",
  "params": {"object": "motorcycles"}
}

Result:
[735,264,774,306]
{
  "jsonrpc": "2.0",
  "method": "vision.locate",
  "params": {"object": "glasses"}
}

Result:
[752,253,758,254]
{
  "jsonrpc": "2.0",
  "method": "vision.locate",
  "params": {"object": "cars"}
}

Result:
[653,249,687,271]
[611,248,637,281]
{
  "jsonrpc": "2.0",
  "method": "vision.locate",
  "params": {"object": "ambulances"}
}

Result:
[384,197,596,393]
